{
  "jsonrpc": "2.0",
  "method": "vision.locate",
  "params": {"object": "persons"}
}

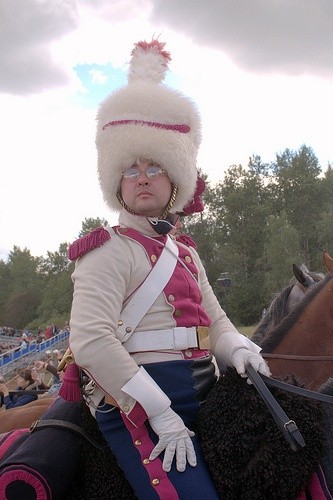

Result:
[0,324,67,410]
[67,158,273,500]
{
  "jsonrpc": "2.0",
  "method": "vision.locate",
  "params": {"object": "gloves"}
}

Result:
[233,348,272,384]
[148,409,199,473]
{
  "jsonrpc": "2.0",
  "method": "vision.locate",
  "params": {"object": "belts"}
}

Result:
[122,324,213,353]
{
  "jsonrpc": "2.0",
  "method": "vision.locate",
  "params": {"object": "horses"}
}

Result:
[0,247,333,499]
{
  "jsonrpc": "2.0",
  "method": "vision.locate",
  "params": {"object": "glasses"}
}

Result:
[120,167,164,183]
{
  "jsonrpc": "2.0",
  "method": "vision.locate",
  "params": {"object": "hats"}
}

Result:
[95,37,203,213]
